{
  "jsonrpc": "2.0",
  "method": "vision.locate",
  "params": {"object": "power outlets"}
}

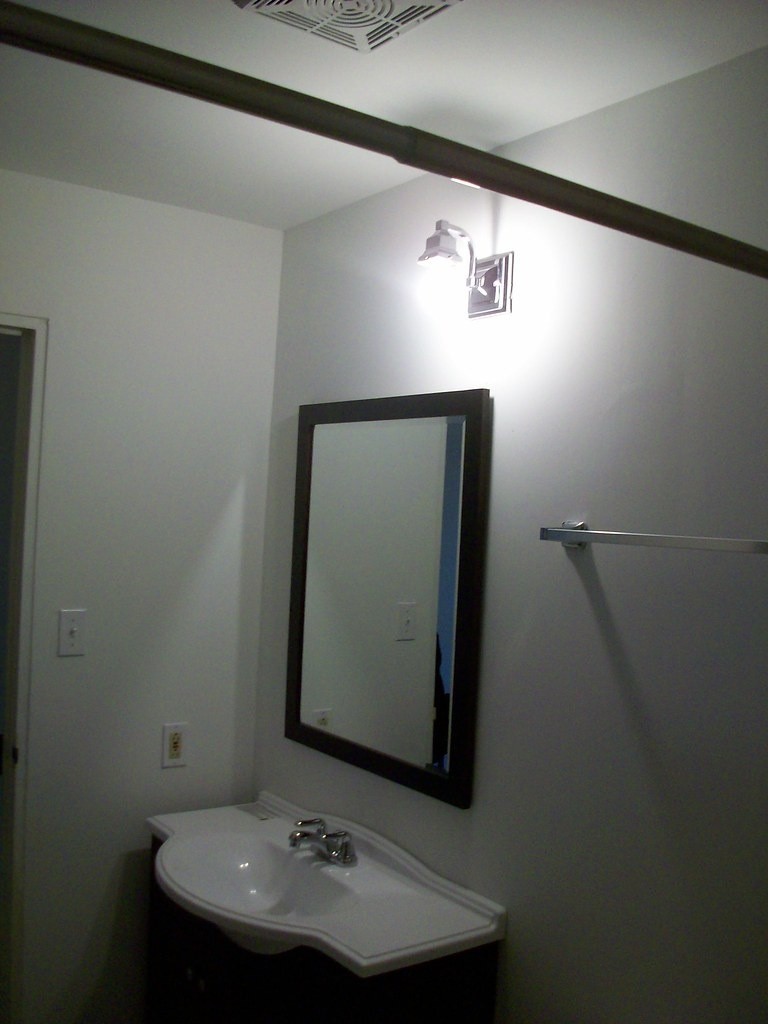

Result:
[162,722,188,768]
[58,610,86,657]
[311,705,335,731]
[395,601,416,644]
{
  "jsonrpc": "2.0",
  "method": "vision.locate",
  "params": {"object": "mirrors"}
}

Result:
[287,387,489,808]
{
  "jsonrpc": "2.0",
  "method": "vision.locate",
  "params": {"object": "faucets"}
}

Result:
[287,830,337,859]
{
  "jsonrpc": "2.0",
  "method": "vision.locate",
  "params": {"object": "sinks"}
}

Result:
[154,821,362,956]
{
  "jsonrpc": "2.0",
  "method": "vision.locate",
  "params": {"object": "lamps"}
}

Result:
[420,221,514,319]
